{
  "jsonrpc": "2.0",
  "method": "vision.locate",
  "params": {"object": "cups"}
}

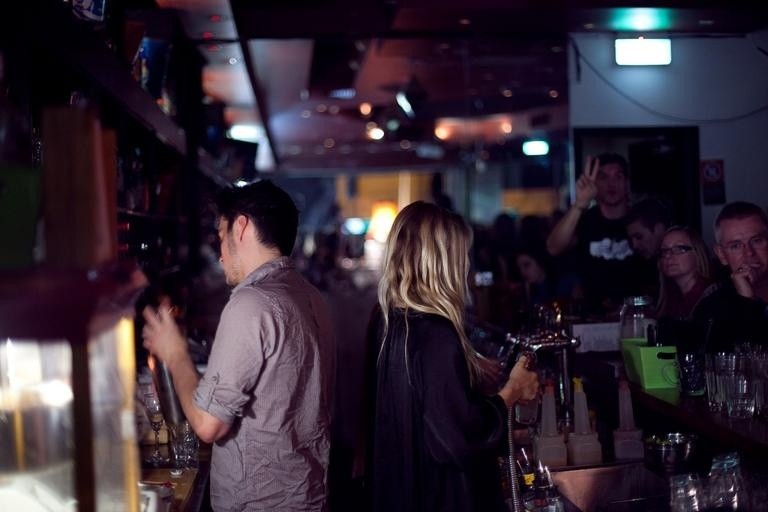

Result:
[661,352,768,419]
[137,481,176,512]
[667,452,749,511]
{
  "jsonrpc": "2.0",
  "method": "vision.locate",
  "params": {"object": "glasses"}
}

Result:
[656,245,695,259]
[718,236,766,251]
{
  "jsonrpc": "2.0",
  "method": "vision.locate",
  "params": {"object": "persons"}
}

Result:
[141,181,337,512]
[363,152,764,508]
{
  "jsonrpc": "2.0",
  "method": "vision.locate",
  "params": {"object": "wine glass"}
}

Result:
[143,393,165,463]
[168,431,199,476]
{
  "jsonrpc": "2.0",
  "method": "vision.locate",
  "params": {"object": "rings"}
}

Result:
[736,267,743,273]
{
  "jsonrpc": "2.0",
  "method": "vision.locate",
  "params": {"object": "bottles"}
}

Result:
[613,381,645,459]
[535,378,567,466]
[152,347,187,425]
[568,378,602,465]
[619,294,657,342]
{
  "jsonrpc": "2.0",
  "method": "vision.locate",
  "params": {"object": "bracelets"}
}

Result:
[575,203,586,211]
